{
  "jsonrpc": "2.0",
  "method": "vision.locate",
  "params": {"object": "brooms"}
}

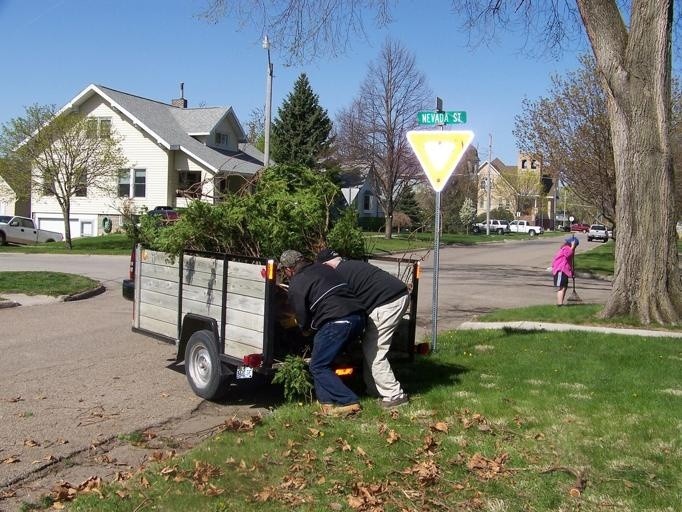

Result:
[567,235,582,301]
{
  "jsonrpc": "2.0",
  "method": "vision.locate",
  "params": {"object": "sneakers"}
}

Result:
[332,403,361,418]
[380,395,409,411]
[312,404,335,417]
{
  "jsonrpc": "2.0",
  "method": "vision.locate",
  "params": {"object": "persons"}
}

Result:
[316,247,410,410]
[552,237,579,306]
[277,248,367,417]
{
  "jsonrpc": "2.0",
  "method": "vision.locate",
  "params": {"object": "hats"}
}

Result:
[317,249,340,264]
[564,237,578,246]
[277,250,304,270]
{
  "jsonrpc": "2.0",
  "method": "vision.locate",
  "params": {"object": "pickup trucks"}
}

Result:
[509,220,544,236]
[0,216,63,245]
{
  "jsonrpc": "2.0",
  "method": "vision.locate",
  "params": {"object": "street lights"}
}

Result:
[263,36,273,167]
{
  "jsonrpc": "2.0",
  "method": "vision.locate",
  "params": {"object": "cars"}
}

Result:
[470,219,511,235]
[123,209,180,301]
[563,223,591,233]
[588,224,612,243]
